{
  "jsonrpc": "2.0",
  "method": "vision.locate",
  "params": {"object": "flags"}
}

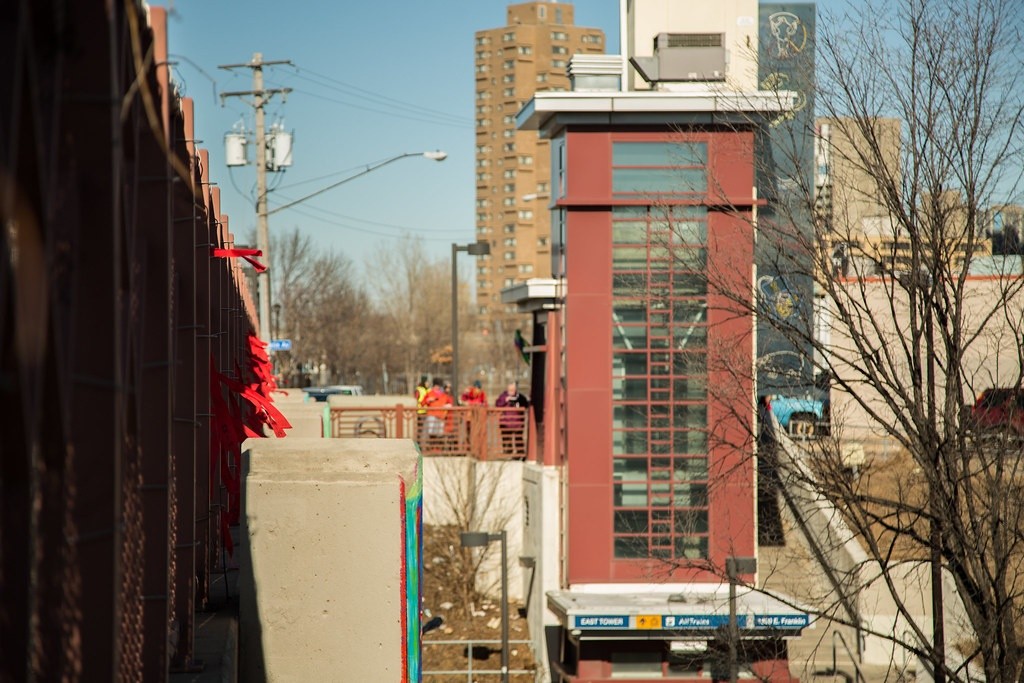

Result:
[515,329,530,367]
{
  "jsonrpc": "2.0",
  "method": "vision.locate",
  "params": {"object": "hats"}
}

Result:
[433,377,443,387]
[422,376,427,383]
[474,380,481,386]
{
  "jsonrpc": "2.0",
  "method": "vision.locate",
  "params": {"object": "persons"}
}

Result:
[415,373,528,460]
[306,377,311,387]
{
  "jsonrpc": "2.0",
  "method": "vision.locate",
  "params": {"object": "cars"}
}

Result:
[758,394,823,435]
[956,387,1024,452]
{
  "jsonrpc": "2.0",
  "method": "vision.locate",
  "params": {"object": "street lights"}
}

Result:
[724,556,755,683]
[458,529,512,683]
[447,241,491,405]
[215,51,449,383]
